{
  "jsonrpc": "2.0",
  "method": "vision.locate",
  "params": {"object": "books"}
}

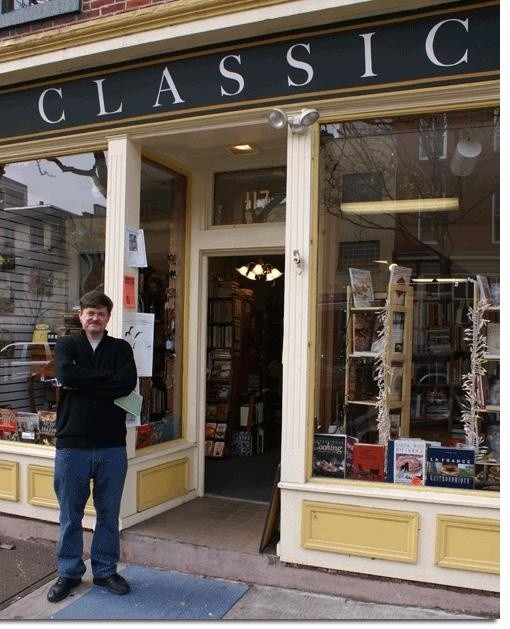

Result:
[347,442,387,481]
[37,409,58,447]
[340,259,500,456]
[0,408,21,442]
[313,431,349,479]
[386,437,426,485]
[204,274,272,458]
[113,388,145,420]
[136,383,180,451]
[422,445,476,488]
[15,412,42,445]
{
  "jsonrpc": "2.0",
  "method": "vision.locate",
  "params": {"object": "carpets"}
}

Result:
[48,564,256,619]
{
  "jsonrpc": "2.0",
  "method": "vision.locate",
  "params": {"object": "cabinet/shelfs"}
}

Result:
[206,298,282,457]
[341,263,500,490]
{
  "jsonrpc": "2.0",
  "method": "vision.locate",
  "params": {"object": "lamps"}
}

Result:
[235,255,284,282]
[267,106,320,135]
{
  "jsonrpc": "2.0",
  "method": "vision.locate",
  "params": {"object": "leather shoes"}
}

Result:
[46,575,84,601]
[93,573,131,595]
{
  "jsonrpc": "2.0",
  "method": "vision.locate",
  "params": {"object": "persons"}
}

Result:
[46,288,132,603]
